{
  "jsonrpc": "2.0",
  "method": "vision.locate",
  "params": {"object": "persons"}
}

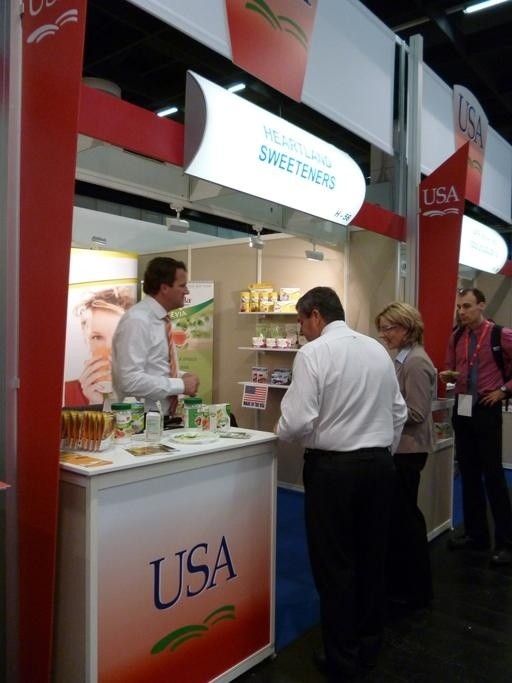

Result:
[111,257,200,417]
[64,287,134,406]
[440,289,512,568]
[375,302,436,618]
[272,287,408,682]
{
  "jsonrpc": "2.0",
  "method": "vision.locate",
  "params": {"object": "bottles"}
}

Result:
[130,402,145,435]
[146,411,162,441]
[201,403,208,429]
[111,403,131,444]
[183,398,203,437]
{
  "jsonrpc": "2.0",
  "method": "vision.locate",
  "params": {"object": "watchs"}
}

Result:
[501,386,509,395]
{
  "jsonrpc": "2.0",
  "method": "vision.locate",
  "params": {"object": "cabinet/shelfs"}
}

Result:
[501,404,512,470]
[52,426,280,683]
[417,397,457,542]
[238,283,299,391]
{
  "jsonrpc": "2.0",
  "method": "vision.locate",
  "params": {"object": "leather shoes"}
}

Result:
[313,648,336,677]
[446,534,492,550]
[489,549,512,564]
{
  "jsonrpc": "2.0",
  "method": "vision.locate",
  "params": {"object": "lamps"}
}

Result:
[306,242,324,263]
[164,205,190,232]
[248,224,266,249]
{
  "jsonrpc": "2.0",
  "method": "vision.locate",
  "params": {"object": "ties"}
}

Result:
[468,334,479,407]
[166,312,179,414]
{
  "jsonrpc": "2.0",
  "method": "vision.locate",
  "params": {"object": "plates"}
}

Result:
[174,432,218,442]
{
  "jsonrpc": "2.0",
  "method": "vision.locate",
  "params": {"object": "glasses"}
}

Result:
[378,325,402,331]
